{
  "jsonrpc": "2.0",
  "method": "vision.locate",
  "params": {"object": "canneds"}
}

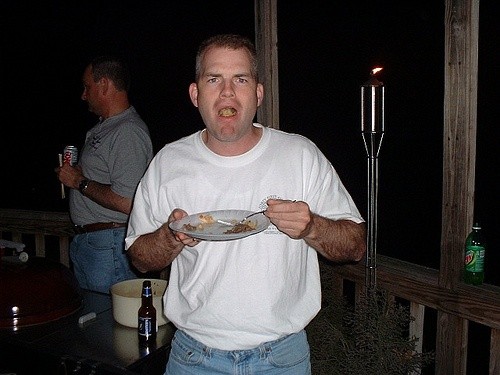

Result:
[63,145,78,167]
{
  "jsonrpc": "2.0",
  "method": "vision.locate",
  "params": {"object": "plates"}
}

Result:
[169,209,270,240]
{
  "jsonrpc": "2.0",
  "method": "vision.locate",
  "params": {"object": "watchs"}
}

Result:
[79,178,90,195]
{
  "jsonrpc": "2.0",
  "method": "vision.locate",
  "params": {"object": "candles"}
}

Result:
[361,67,384,87]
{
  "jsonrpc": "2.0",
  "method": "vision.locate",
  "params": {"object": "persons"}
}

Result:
[125,34,366,375]
[54,55,154,294]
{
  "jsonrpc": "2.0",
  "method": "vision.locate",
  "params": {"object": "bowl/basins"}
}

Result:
[110,279,170,328]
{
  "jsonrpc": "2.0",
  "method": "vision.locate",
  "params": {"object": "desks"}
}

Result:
[0,287,177,375]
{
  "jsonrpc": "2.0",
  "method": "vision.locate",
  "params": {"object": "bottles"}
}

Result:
[462,222,485,286]
[137,280,157,344]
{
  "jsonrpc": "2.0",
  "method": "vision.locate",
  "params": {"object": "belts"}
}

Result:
[73,222,126,235]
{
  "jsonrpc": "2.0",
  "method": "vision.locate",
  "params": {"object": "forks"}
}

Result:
[217,200,296,227]
